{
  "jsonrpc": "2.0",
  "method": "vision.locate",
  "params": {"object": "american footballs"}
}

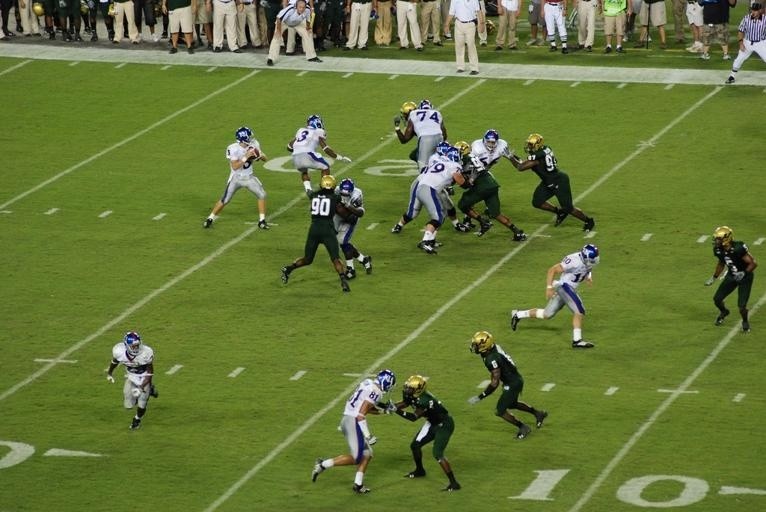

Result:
[248,147,260,161]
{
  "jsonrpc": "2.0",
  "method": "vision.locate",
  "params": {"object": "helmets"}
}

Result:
[32,3,44,16]
[307,115,323,129]
[581,244,600,265]
[124,332,141,356]
[320,175,336,190]
[469,331,494,354]
[436,141,469,163]
[340,179,354,194]
[712,226,734,253]
[400,102,416,121]
[483,130,498,144]
[235,127,254,145]
[80,1,89,14]
[403,375,426,399]
[524,134,544,153]
[418,100,432,109]
[376,370,396,392]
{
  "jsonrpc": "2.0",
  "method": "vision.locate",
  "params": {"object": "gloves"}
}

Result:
[394,117,401,131]
[107,376,114,383]
[467,396,480,403]
[733,272,745,281]
[382,400,397,414]
[704,275,717,286]
[337,155,352,163]
[131,389,143,397]
[243,151,255,163]
[502,147,513,159]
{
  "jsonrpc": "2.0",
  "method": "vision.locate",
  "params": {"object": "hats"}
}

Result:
[750,3,762,11]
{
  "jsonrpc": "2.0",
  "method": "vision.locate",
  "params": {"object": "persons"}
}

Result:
[279,115,373,290]
[389,97,596,254]
[202,127,278,235]
[468,328,548,441]
[387,373,462,494]
[104,332,160,432]
[1,0,522,76]
[702,224,757,333]
[725,0,766,83]
[312,369,394,494]
[527,0,739,61]
[510,244,600,349]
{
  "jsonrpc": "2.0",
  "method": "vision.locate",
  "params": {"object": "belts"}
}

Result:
[456,18,474,23]
[549,3,558,6]
[354,2,370,4]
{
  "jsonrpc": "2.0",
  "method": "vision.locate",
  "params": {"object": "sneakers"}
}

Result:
[536,411,547,428]
[686,44,710,60]
[583,218,594,233]
[353,483,370,494]
[342,47,349,51]
[312,458,326,481]
[554,209,567,227]
[742,321,750,332]
[281,266,288,283]
[203,218,213,228]
[340,256,372,291]
[366,435,376,444]
[517,426,530,440]
[404,470,425,477]
[0,26,98,41]
[725,76,735,83]
[513,230,527,241]
[511,310,519,330]
[442,481,460,491]
[109,33,160,43]
[417,237,442,256]
[550,44,627,54]
[391,224,402,233]
[267,59,272,64]
[308,57,322,62]
[258,220,270,230]
[362,47,369,50]
[572,339,593,347]
[723,54,731,60]
[129,417,140,430]
[162,31,262,54]
[150,385,158,398]
[453,217,493,236]
[716,310,729,325]
